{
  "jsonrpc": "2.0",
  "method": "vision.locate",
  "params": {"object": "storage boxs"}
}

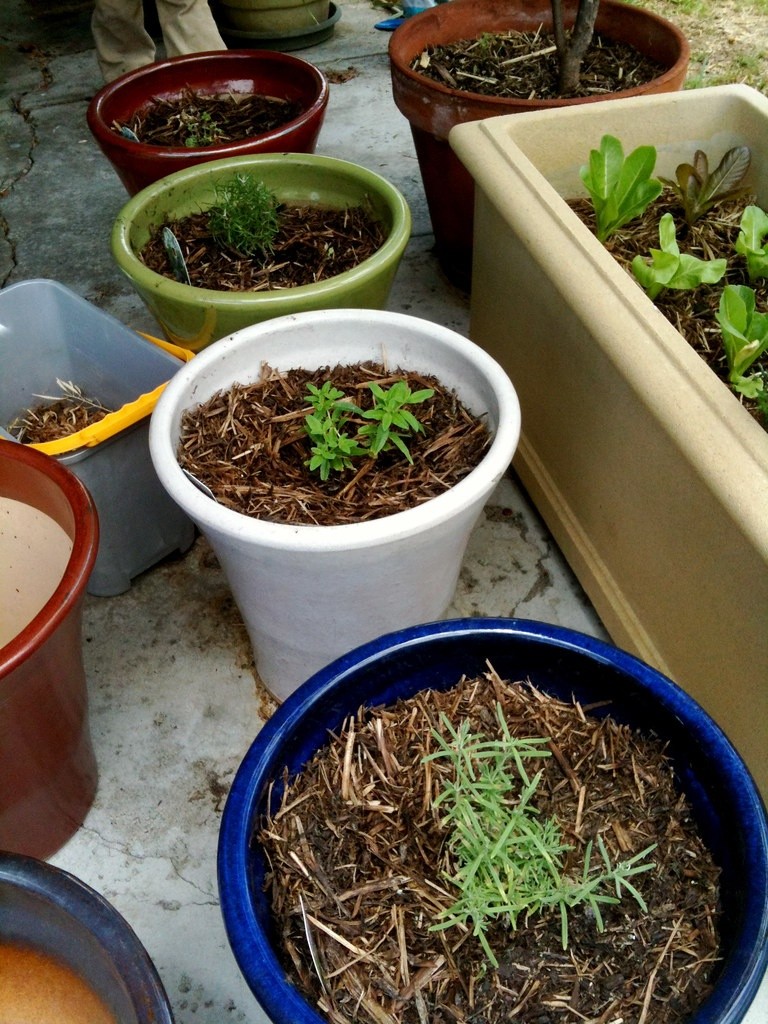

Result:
[1,280,193,595]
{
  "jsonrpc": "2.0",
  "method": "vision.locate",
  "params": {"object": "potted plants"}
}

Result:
[446,81,768,809]
[85,50,329,199]
[215,611,768,1024]
[145,306,523,707]
[109,151,414,370]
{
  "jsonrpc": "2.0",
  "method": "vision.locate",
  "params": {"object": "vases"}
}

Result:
[388,0,691,304]
[225,0,340,51]
[0,439,97,864]
[0,849,174,1024]
